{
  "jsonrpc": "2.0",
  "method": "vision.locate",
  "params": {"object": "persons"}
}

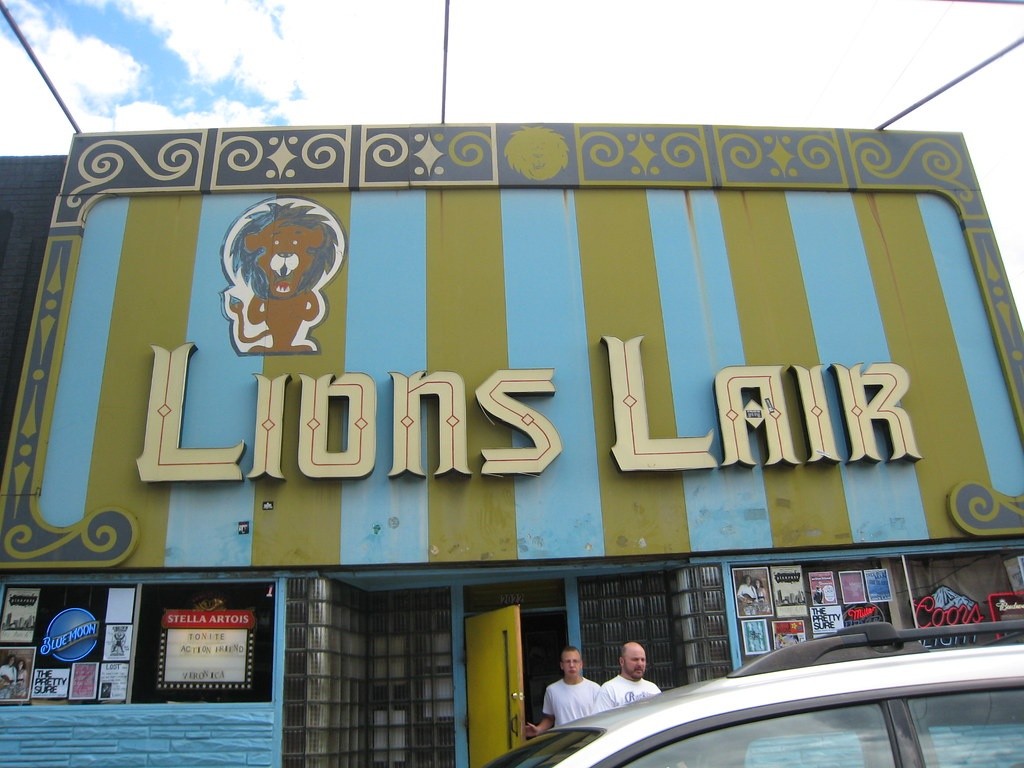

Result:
[524,646,600,737]
[0,654,29,699]
[596,642,662,714]
[737,575,771,615]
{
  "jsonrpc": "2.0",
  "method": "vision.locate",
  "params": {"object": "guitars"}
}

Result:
[739,593,757,607]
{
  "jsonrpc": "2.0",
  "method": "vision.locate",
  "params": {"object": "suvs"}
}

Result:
[486,617,1024,768]
